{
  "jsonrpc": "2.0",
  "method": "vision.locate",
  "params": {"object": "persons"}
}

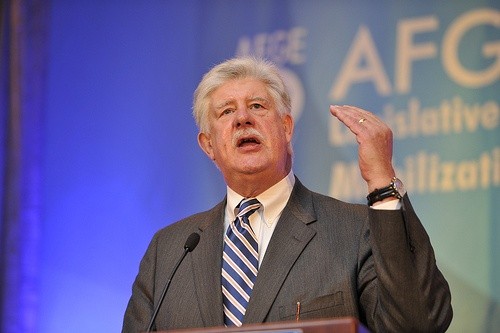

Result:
[121,52,453,333]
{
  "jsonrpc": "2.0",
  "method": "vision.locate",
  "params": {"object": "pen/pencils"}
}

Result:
[296,302,300,320]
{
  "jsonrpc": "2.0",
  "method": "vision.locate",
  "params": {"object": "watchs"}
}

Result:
[365,176,407,207]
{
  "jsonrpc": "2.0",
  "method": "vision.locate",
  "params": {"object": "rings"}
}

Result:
[358,119,367,124]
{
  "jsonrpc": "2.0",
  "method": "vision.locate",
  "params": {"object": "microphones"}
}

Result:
[145,233,200,333]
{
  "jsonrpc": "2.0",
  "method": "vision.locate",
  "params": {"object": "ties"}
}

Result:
[220,198,261,328]
